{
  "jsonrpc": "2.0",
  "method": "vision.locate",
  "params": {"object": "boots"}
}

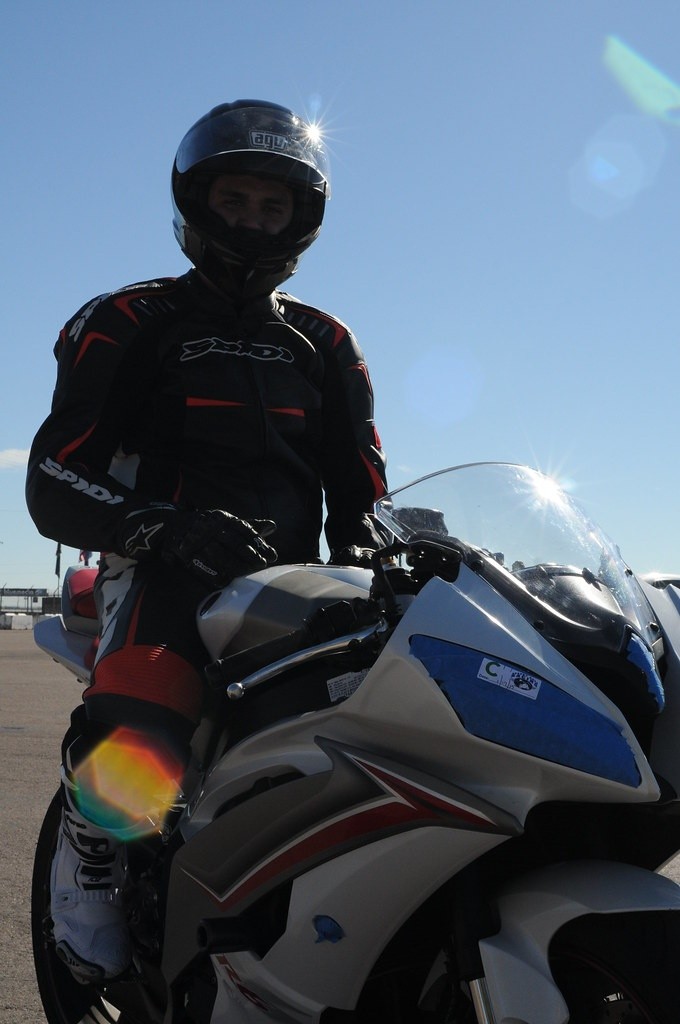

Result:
[52,764,161,984]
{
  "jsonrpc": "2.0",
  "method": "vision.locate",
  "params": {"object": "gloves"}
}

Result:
[330,545,397,569]
[117,501,278,589]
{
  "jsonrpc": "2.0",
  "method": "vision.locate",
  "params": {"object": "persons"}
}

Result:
[27,100,392,980]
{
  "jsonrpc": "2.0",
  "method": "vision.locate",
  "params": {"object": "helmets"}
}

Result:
[171,99,332,301]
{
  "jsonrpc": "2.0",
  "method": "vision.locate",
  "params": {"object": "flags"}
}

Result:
[55,542,61,579]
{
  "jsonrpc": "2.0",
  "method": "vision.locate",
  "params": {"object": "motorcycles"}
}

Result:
[32,462,680,1024]
